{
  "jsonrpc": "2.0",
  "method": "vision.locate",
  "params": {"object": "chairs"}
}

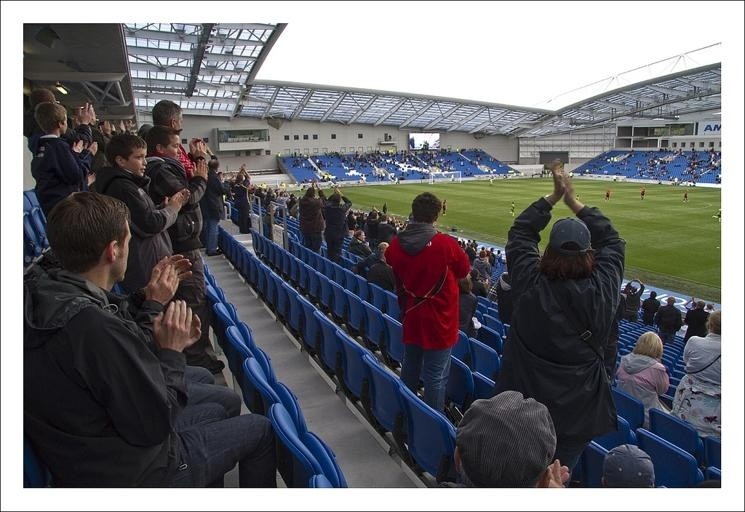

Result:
[24,148,721,488]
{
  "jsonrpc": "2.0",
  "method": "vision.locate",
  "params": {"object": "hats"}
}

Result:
[550,217,595,255]
[456,391,557,487]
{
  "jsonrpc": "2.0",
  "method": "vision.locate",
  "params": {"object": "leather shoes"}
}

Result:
[206,249,222,256]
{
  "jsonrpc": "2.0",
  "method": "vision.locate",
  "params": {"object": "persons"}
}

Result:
[23,87,347,420]
[437,389,570,488]
[384,192,470,413]
[504,158,625,480]
[23,191,277,488]
[346,223,516,340]
[347,136,721,222]
[621,278,721,488]
